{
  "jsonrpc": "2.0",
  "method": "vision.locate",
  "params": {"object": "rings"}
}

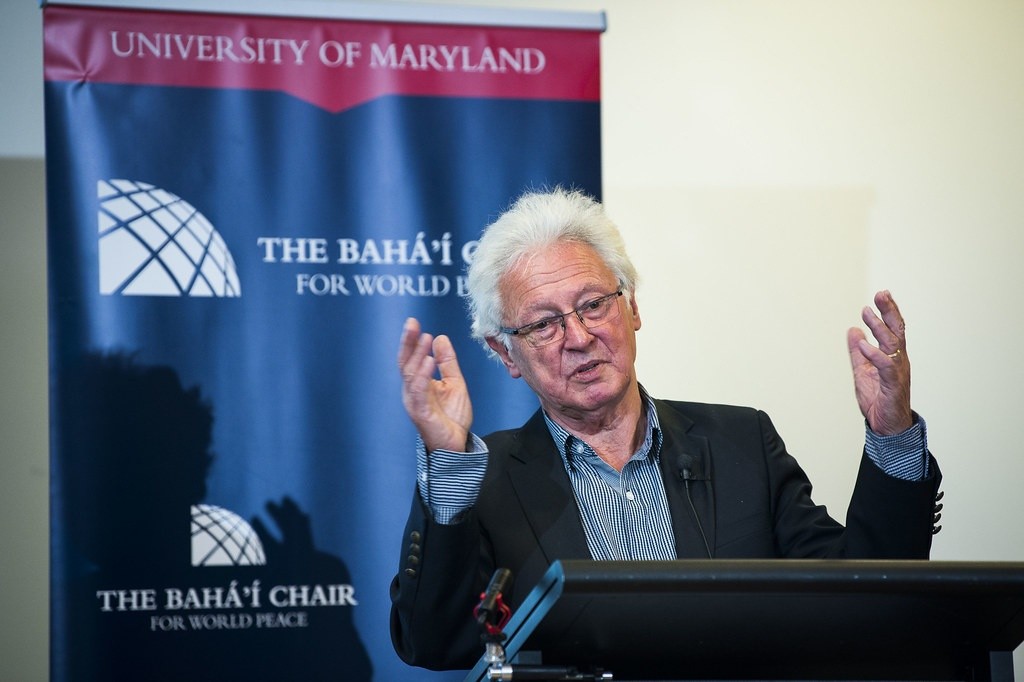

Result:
[887,349,901,358]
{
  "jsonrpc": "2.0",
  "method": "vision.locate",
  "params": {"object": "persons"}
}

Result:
[388,185,942,673]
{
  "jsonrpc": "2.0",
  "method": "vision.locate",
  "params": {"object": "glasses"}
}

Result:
[497,279,624,348]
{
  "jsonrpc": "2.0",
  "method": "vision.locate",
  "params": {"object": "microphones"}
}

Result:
[678,454,693,481]
[477,567,515,626]
